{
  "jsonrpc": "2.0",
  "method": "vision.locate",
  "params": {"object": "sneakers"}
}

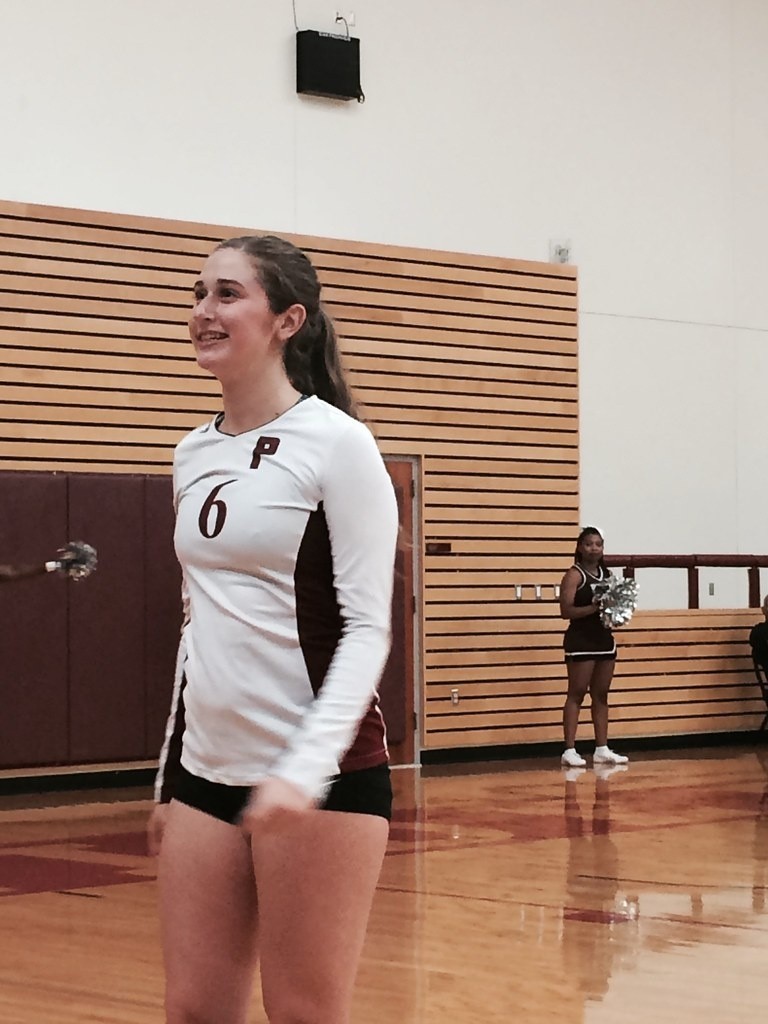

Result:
[561,748,587,766]
[592,746,629,764]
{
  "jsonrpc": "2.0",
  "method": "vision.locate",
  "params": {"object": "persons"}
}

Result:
[148,234,399,1024]
[749,595,768,680]
[560,528,630,766]
